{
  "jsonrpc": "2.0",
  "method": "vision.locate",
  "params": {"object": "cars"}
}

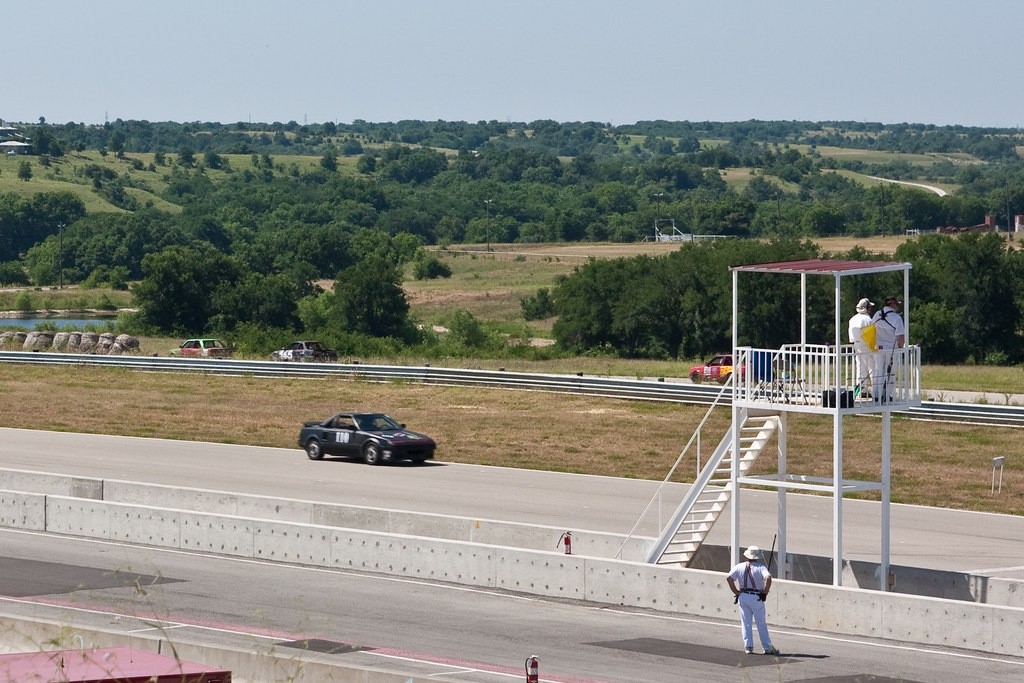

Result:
[168,338,233,360]
[269,340,337,363]
[298,412,437,465]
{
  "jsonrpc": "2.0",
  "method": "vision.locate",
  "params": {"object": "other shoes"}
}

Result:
[860,393,893,402]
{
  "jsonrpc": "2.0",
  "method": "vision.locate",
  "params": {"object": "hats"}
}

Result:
[884,296,901,305]
[858,298,875,306]
[743,546,760,559]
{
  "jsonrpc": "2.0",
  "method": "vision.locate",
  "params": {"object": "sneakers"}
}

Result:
[745,648,752,653]
[763,648,779,653]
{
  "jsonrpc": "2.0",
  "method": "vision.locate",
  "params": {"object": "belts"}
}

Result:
[742,591,760,595]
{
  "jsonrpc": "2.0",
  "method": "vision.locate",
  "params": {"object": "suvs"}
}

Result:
[689,354,753,385]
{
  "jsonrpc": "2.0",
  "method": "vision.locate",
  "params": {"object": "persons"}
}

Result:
[870,299,908,403]
[848,298,881,397]
[725,545,780,654]
